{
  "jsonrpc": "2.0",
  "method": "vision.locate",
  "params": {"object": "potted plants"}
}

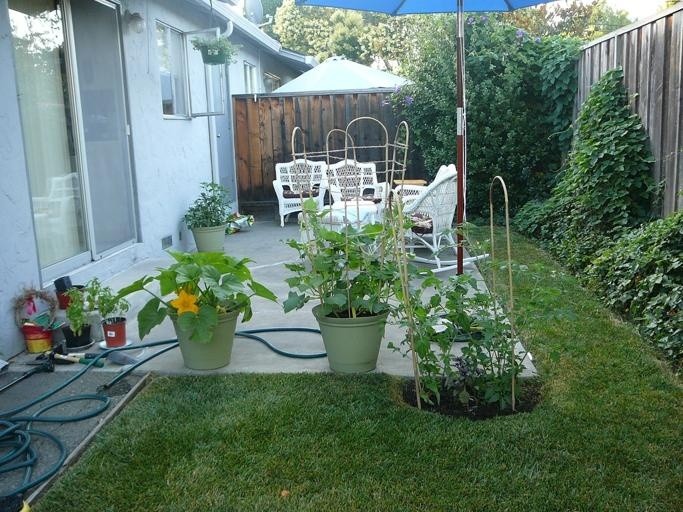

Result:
[183,181,232,251]
[190,34,244,66]
[283,198,415,375]
[86,277,130,347]
[61,286,91,347]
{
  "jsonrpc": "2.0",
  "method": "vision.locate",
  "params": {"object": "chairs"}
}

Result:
[325,159,390,201]
[272,157,328,226]
[401,171,458,268]
[393,163,456,204]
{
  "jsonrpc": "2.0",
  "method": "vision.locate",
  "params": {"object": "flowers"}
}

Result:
[111,250,278,344]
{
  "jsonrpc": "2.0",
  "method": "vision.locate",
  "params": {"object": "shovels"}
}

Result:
[62,351,139,365]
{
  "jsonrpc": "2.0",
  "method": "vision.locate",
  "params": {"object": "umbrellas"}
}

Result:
[295,0,553,271]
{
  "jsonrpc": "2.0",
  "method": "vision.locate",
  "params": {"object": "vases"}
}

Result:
[167,311,239,370]
[56,285,85,309]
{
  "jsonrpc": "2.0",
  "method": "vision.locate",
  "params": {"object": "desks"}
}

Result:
[298,199,389,254]
[394,179,427,186]
